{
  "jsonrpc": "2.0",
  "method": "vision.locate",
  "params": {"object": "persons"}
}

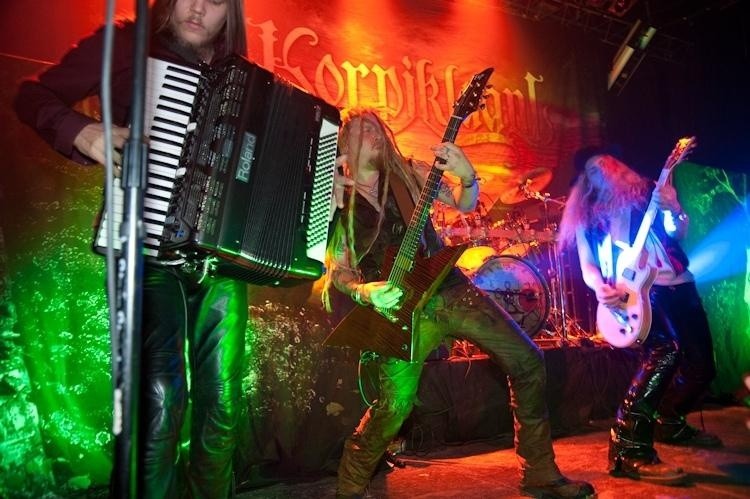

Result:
[320,105,596,499]
[447,200,493,247]
[553,143,723,485]
[10,2,357,498]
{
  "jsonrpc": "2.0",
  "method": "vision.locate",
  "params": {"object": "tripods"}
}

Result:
[447,200,590,361]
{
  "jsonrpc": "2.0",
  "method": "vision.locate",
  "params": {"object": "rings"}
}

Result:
[446,154,449,161]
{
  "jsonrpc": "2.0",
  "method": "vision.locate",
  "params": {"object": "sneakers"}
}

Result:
[609,453,687,485]
[519,477,596,498]
[653,423,721,450]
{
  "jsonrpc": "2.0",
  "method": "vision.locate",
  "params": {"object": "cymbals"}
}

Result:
[523,197,567,222]
[500,168,554,204]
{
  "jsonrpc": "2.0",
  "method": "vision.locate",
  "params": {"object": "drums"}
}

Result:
[444,212,532,274]
[472,255,551,340]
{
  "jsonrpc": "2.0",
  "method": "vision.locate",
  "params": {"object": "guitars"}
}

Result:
[596,136,697,348]
[321,68,496,363]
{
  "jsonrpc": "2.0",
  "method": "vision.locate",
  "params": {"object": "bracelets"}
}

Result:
[460,172,480,189]
[349,284,370,308]
